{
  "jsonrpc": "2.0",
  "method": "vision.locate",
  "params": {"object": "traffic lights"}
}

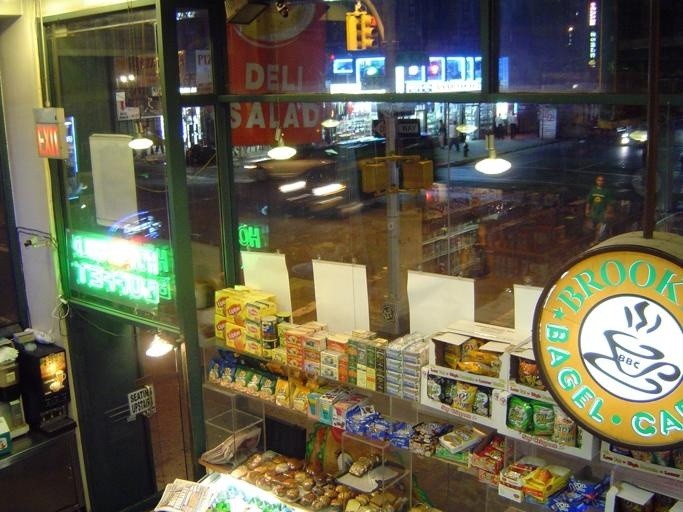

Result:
[356,13,379,52]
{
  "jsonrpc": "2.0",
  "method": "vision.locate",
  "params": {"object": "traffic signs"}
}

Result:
[371,118,421,140]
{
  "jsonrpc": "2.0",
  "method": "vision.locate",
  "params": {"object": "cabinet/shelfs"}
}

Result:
[198,338,683,512]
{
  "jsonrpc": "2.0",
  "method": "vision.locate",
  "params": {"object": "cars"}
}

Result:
[249,131,437,214]
[596,99,683,148]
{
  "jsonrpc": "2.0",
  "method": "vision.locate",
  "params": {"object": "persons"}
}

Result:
[435,117,446,149]
[584,175,612,249]
[494,112,505,140]
[507,111,518,140]
[446,119,459,151]
[597,201,623,244]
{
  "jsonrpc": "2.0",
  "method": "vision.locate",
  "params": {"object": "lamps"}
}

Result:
[264,1,298,162]
[473,102,512,175]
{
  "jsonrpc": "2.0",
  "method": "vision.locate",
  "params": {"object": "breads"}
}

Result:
[232,454,442,512]
